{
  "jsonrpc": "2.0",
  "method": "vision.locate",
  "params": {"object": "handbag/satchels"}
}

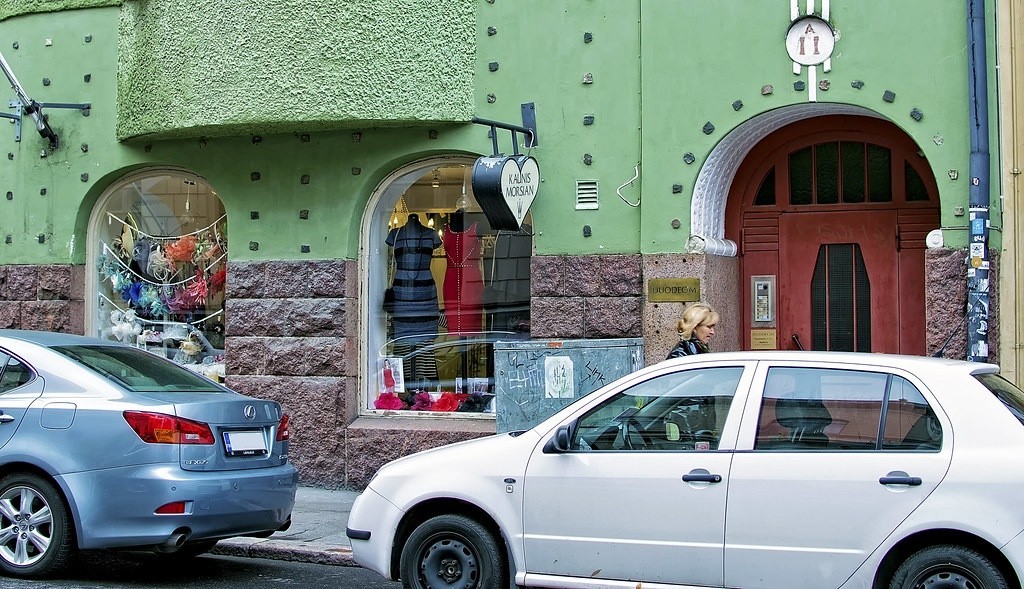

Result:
[382,287,397,314]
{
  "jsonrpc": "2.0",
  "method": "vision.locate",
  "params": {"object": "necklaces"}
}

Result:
[404,222,422,279]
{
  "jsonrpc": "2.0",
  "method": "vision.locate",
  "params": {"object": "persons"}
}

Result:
[667,303,719,433]
[384,213,442,341]
[495,223,532,315]
[382,359,396,392]
[442,209,484,336]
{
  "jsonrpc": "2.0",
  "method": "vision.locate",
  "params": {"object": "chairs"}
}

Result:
[769,391,814,449]
[791,400,832,448]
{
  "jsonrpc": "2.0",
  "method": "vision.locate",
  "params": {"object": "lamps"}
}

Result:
[455,168,471,211]
[179,184,195,226]
[431,168,440,188]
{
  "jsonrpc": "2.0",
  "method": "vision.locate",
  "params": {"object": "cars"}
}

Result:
[0,326,300,581]
[371,330,531,395]
[347,350,1024,589]
[132,318,225,365]
